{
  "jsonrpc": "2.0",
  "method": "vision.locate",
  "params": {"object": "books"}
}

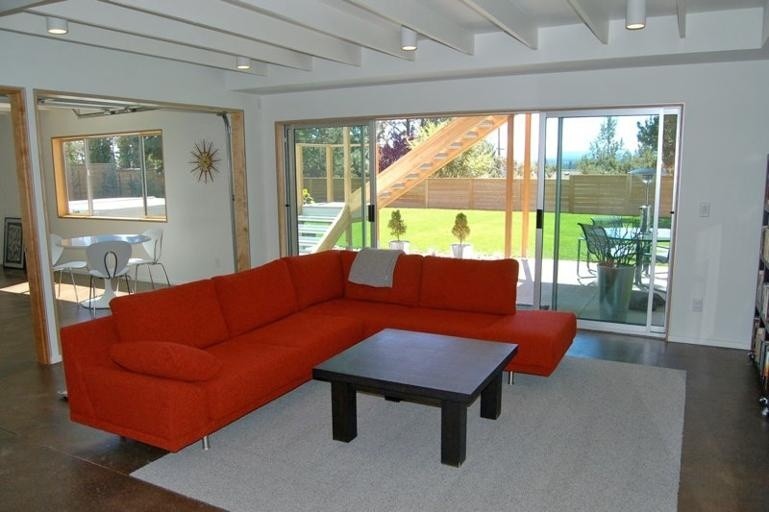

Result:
[752,198,769,390]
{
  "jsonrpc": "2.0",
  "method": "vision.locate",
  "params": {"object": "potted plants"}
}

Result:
[450,211,472,259]
[386,209,410,255]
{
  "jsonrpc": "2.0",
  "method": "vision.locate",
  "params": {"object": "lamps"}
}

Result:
[47,15,68,35]
[625,0,647,31]
[400,26,417,51]
[236,56,250,70]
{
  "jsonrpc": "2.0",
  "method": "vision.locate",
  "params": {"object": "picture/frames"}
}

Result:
[3,216,25,269]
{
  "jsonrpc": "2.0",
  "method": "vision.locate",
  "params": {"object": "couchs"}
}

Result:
[59,249,577,454]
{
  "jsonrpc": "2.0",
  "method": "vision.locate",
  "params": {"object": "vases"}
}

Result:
[596,262,635,323]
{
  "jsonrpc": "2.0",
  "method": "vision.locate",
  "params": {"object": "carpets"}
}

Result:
[128,355,687,512]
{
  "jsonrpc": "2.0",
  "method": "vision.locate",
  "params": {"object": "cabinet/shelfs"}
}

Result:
[749,154,769,403]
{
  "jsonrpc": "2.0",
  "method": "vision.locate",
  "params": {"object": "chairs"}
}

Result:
[49,227,171,322]
[577,215,671,297]
